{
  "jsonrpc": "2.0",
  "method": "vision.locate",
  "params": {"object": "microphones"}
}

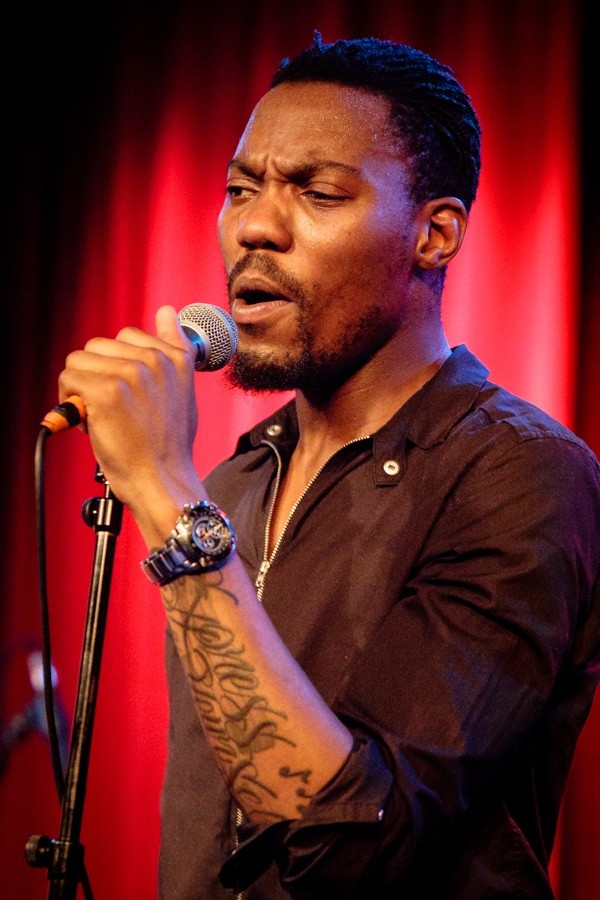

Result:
[39,304,240,435]
[25,645,68,779]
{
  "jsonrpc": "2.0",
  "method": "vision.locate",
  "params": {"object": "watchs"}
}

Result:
[140,500,239,587]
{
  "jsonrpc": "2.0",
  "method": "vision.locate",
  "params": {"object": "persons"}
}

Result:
[59,36,600,900]
